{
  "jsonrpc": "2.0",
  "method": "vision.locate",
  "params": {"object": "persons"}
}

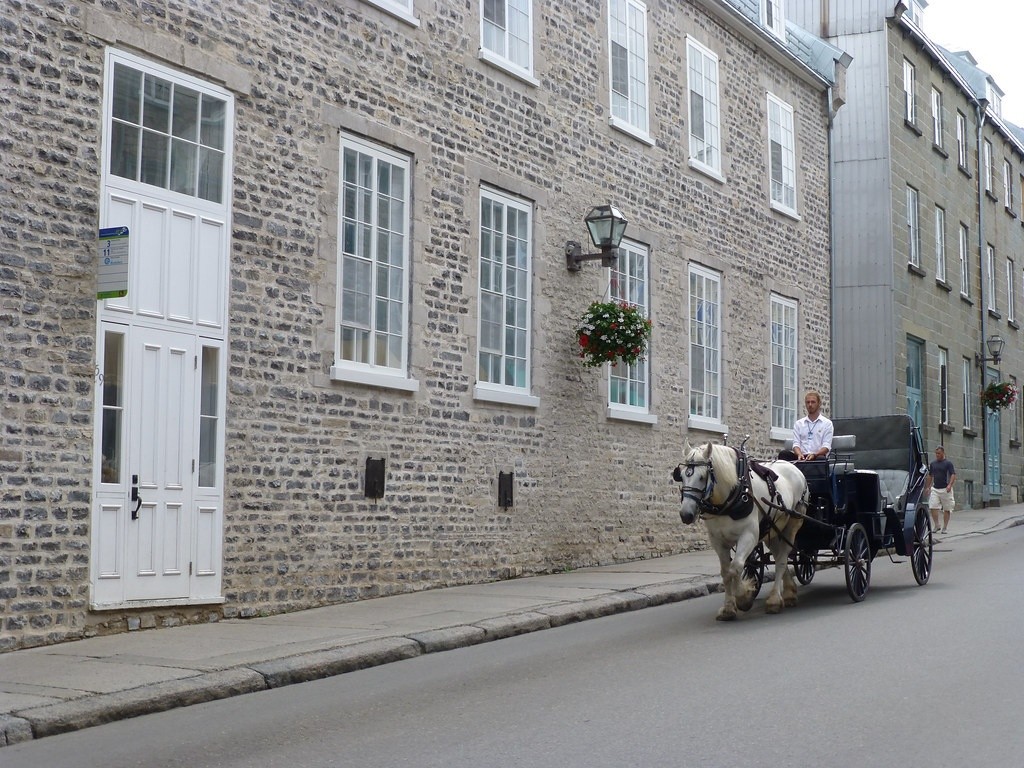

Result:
[776,389,835,462]
[925,443,957,535]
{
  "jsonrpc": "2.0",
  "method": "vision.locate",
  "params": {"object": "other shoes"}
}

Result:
[941,528,948,534]
[932,525,941,533]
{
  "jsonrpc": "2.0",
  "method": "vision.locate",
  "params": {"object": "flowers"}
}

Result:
[983,365,1020,411]
[572,264,654,371]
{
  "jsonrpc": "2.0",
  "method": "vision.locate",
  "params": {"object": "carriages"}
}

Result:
[672,413,933,622]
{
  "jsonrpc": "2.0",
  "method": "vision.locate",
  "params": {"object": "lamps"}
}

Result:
[584,205,629,248]
[986,335,1006,356]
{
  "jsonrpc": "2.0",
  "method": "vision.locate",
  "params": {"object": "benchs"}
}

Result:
[868,469,910,513]
[827,434,857,514]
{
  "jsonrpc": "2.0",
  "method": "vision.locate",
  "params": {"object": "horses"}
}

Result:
[678,437,811,623]
